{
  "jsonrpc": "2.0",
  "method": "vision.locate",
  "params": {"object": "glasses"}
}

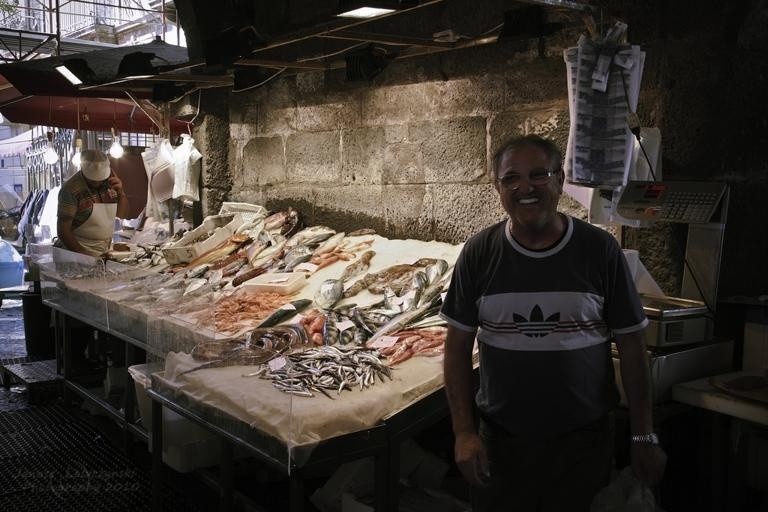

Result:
[494,170,562,190]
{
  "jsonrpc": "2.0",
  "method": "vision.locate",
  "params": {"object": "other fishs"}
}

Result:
[88,204,455,400]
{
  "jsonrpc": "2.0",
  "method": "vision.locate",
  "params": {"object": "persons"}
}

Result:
[48,150,129,377]
[439,136,667,512]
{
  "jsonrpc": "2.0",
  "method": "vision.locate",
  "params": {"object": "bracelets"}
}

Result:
[630,433,660,445]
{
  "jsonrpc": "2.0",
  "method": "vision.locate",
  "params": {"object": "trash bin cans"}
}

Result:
[20,292,56,355]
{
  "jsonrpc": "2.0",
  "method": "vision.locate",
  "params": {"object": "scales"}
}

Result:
[617,180,729,346]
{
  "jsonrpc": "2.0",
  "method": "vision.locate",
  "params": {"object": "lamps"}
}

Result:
[204,23,263,67]
[338,46,396,81]
[491,5,558,47]
[41,107,60,168]
[66,98,87,170]
[109,48,177,83]
[106,90,125,161]
[50,54,96,89]
[146,82,198,103]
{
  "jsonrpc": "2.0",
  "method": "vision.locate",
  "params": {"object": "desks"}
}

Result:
[37,299,176,455]
[137,382,486,511]
[679,365,765,512]
[604,405,722,511]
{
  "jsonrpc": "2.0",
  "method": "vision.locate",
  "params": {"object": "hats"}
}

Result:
[81,149,112,182]
